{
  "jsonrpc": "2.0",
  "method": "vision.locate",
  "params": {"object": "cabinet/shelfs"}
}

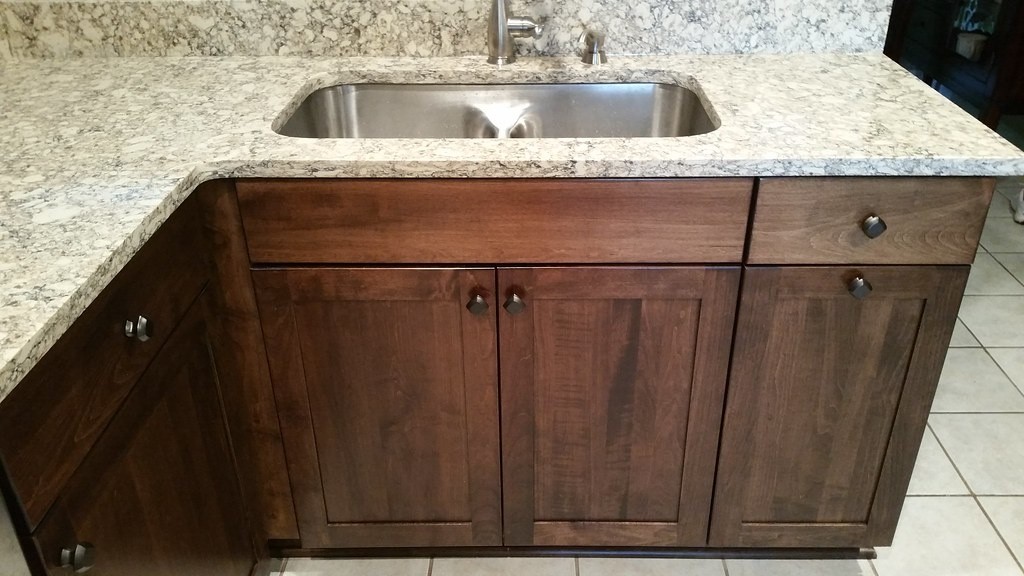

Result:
[0,176,1003,576]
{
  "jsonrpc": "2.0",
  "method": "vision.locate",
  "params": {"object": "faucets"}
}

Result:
[486,0,544,65]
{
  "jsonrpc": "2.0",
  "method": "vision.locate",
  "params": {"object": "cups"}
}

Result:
[956,34,987,63]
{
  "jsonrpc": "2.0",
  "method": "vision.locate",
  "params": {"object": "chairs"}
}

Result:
[922,0,1013,132]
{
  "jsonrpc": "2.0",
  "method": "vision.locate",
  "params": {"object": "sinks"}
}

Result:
[271,65,723,138]
